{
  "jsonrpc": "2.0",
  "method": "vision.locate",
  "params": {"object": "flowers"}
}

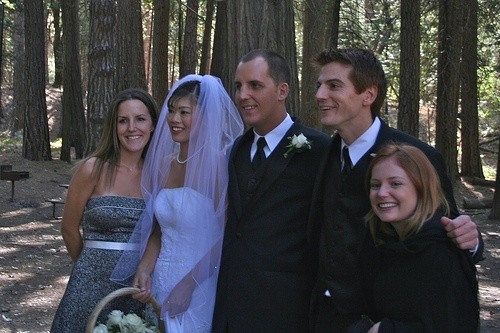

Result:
[282,133,312,158]
[93,310,160,333]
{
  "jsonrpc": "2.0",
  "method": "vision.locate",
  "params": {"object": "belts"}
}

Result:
[316,294,338,304]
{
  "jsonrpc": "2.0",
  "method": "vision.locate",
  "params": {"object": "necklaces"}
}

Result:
[176,141,206,164]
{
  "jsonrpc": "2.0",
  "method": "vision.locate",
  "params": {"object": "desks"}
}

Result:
[0,165,30,202]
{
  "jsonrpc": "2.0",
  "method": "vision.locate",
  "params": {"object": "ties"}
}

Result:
[342,146,352,177]
[251,137,267,170]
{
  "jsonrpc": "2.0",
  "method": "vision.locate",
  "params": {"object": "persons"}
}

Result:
[307,44,486,333]
[211,50,333,333]
[109,75,245,333]
[49,89,160,333]
[349,143,480,333]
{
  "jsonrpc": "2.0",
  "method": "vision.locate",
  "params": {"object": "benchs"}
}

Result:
[45,199,64,221]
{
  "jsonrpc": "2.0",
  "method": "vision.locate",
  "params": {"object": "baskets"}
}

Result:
[84,287,165,333]
